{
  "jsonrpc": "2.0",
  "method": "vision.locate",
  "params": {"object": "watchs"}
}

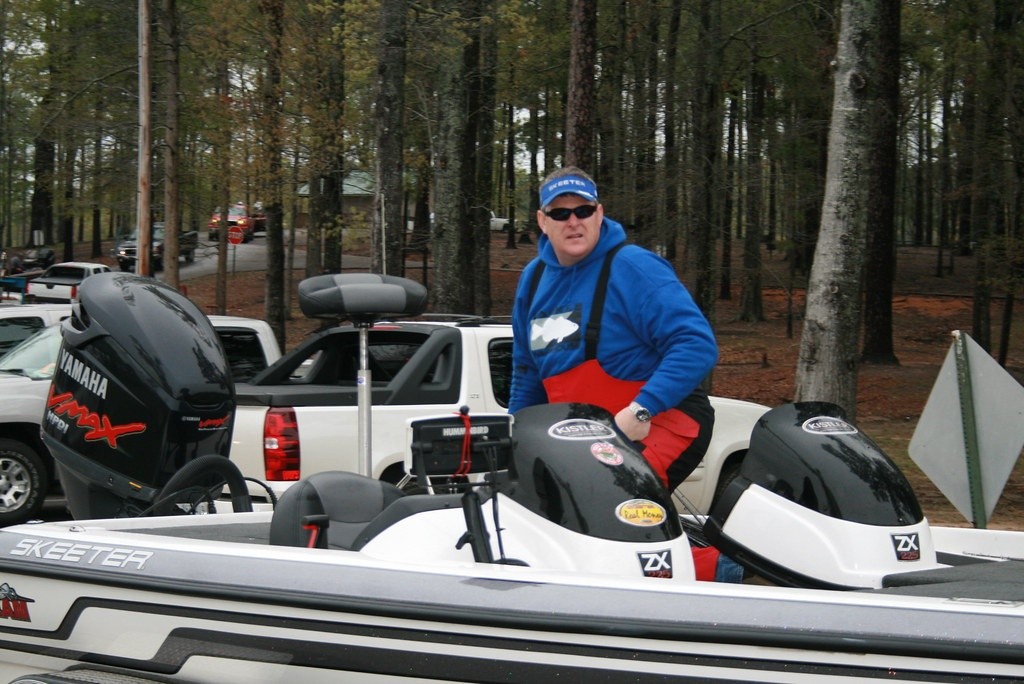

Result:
[628,402,652,423]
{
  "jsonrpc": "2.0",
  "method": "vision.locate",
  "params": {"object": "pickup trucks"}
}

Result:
[25,260,113,305]
[114,221,198,277]
[0,273,1024,684]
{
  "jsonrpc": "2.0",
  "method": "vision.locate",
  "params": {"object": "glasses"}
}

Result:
[545,204,597,221]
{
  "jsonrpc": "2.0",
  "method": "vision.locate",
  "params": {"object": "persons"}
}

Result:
[507,166,757,583]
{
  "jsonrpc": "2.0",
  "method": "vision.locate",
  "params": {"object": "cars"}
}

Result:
[208,201,266,242]
[489,210,519,233]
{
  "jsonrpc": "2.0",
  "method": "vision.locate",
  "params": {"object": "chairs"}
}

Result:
[269,470,408,550]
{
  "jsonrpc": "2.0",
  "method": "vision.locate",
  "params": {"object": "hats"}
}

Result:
[540,175,597,209]
